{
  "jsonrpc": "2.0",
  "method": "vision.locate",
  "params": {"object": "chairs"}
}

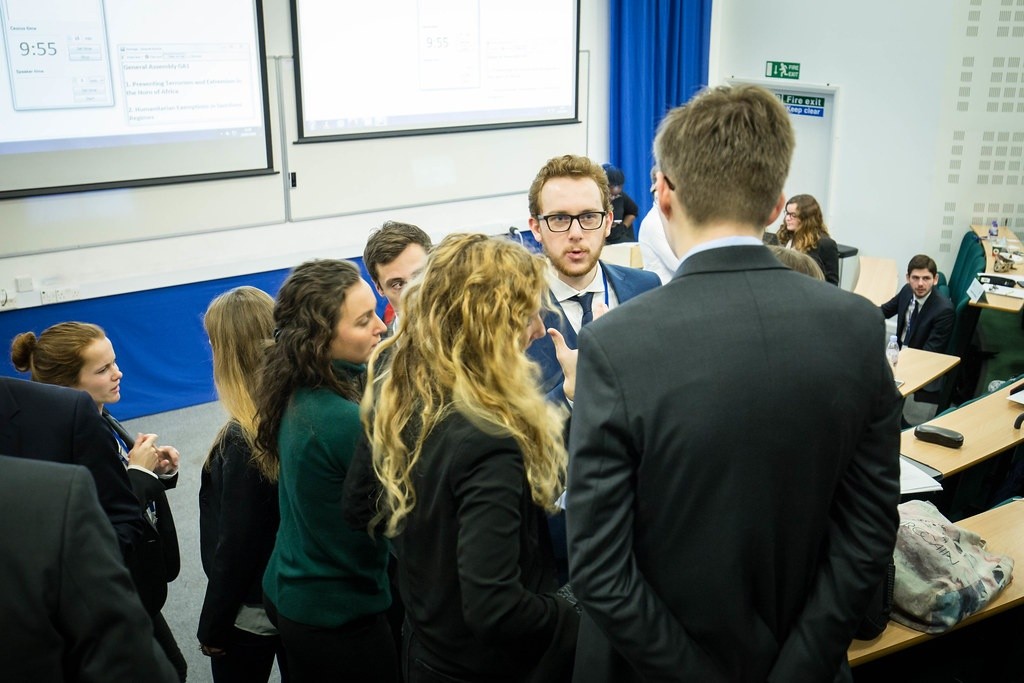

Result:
[936,231,986,354]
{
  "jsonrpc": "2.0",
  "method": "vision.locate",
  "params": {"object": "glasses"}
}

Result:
[536,212,608,232]
[784,209,799,219]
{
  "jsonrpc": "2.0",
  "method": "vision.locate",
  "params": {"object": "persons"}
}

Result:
[0,455,181,683]
[357,232,580,682]
[776,193,840,286]
[521,154,661,432]
[571,85,902,683]
[602,163,638,244]
[362,220,435,421]
[11,320,185,580]
[878,254,955,428]
[764,245,825,282]
[246,260,395,683]
[637,165,679,287]
[194,287,293,683]
[1,376,188,683]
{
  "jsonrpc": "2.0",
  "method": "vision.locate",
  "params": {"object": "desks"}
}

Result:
[900,377,1024,481]
[847,498,1024,667]
[897,348,961,398]
[853,256,898,307]
[969,225,1024,313]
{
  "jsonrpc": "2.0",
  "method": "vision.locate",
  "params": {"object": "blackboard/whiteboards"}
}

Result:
[278,49,589,223]
[0,55,288,259]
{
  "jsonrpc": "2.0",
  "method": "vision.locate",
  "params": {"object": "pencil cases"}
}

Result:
[914,424,964,448]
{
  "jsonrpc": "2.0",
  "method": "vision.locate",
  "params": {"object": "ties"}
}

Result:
[569,292,594,328]
[910,300,919,331]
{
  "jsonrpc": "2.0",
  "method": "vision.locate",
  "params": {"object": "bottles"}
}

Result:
[989,219,998,244]
[887,335,899,380]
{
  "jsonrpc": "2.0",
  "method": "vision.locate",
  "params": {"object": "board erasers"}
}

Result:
[291,172,296,188]
